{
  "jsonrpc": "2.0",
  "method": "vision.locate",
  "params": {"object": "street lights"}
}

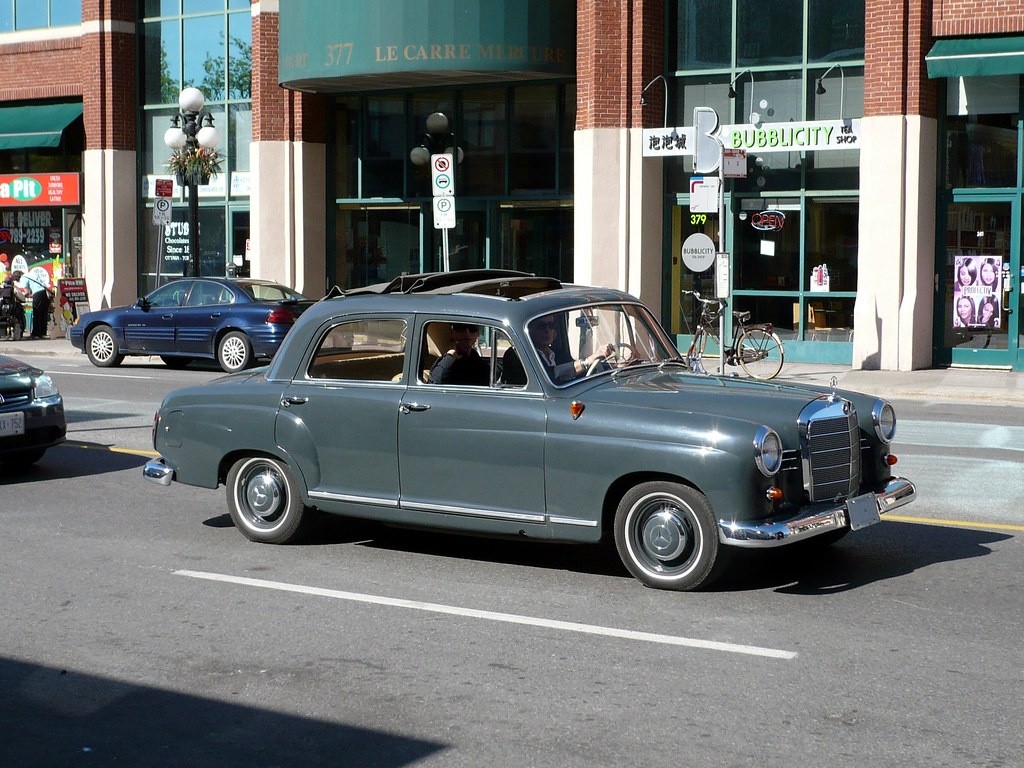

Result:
[411,110,469,274]
[164,86,219,279]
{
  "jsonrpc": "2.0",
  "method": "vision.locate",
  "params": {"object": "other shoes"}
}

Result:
[27,335,43,340]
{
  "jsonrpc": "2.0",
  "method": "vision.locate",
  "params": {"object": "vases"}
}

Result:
[175,166,210,186]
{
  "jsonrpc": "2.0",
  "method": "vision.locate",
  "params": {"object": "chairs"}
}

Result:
[391,322,455,383]
[792,303,832,342]
[201,294,220,305]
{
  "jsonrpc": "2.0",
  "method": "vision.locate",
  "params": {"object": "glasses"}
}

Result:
[454,323,478,333]
[537,321,555,329]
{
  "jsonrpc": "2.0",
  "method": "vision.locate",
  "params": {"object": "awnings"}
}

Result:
[0,102,83,150]
[925,34,1024,79]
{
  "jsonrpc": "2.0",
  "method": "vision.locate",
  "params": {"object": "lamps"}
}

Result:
[638,75,668,128]
[727,69,754,124]
[816,63,844,120]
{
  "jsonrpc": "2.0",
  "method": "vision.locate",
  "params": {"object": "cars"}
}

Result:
[142,269,920,590]
[0,351,69,469]
[69,277,325,375]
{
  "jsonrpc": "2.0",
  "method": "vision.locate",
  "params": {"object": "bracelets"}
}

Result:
[580,357,588,371]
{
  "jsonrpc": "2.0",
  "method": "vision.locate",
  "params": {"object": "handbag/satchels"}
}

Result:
[45,287,55,299]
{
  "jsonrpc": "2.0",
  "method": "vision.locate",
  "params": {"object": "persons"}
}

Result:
[980,258,998,291]
[955,295,975,327]
[428,322,490,386]
[977,294,999,327]
[954,258,977,291]
[0,261,50,340]
[501,314,615,384]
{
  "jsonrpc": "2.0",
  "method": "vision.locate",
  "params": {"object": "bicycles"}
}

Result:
[681,287,785,381]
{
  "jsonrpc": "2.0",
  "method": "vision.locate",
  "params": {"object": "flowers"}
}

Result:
[160,145,229,182]
